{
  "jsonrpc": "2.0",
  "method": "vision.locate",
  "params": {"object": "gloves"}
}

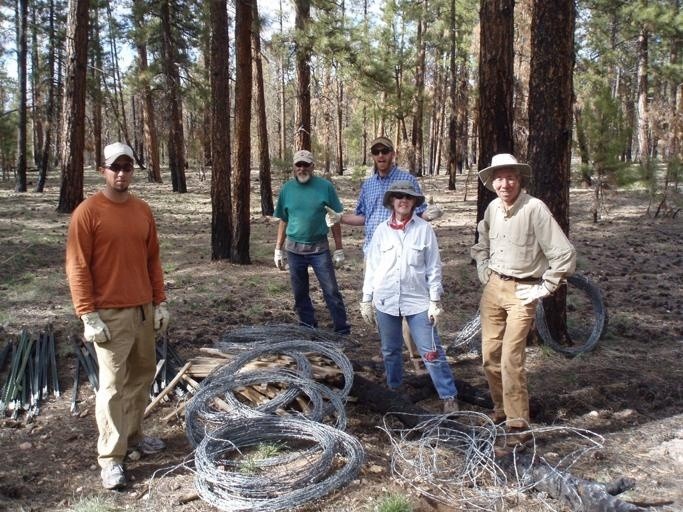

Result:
[274,249,284,270]
[428,301,444,326]
[323,206,343,227]
[358,300,374,327]
[332,249,344,269]
[81,312,111,344]
[516,283,551,306]
[153,301,168,331]
[477,260,491,285]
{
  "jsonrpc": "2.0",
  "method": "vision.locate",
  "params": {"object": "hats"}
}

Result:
[102,141,135,165]
[479,154,531,193]
[383,180,424,209]
[371,137,393,149]
[294,150,313,164]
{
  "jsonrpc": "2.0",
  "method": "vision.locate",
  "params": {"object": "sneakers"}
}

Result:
[508,428,530,445]
[444,397,458,418]
[128,436,165,454]
[480,413,506,427]
[101,464,126,489]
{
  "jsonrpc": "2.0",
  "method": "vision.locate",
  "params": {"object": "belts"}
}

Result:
[495,272,540,282]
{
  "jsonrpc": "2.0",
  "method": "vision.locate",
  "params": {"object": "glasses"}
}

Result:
[104,162,132,173]
[295,163,310,168]
[372,149,391,156]
[392,193,415,200]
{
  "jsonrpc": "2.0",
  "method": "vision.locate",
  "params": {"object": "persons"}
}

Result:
[271,151,347,335]
[65,141,168,491]
[469,151,577,447]
[323,137,442,359]
[359,181,459,419]
